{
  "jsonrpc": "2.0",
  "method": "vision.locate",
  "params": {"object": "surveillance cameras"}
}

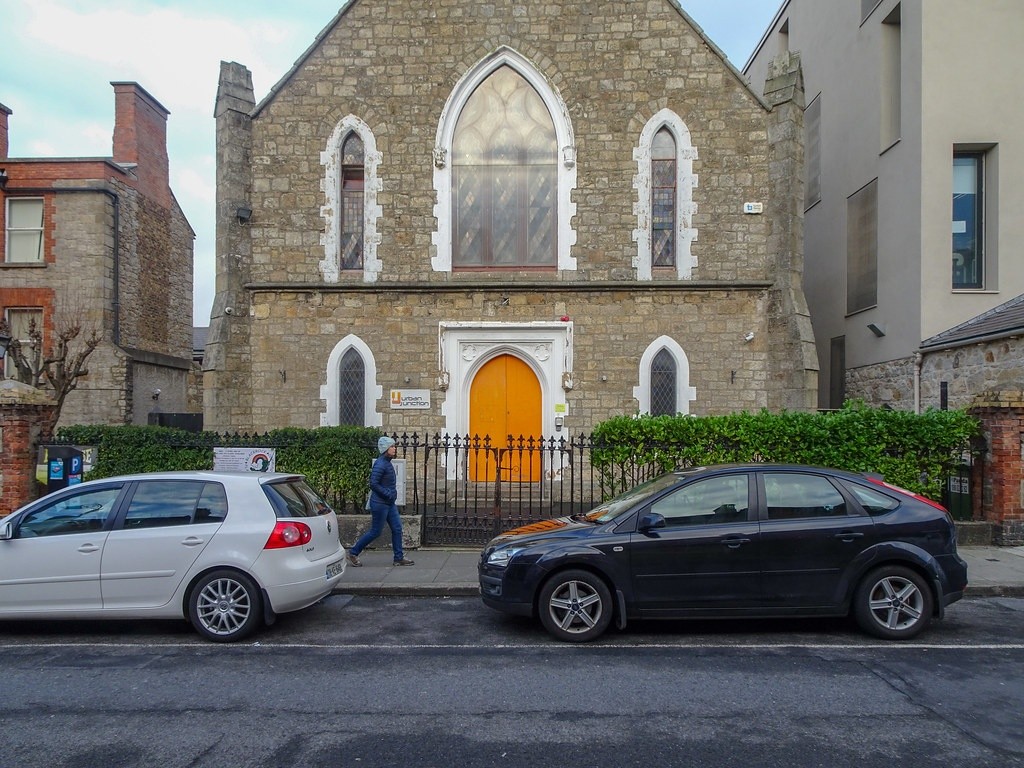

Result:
[744,334,754,342]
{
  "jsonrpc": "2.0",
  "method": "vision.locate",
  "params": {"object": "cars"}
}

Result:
[476,462,970,642]
[0,470,351,642]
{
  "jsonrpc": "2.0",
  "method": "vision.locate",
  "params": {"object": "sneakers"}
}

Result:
[394,559,415,566]
[347,554,362,567]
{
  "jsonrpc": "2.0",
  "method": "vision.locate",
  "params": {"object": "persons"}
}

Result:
[345,437,415,567]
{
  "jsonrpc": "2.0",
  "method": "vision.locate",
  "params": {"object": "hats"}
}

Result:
[378,436,395,454]
